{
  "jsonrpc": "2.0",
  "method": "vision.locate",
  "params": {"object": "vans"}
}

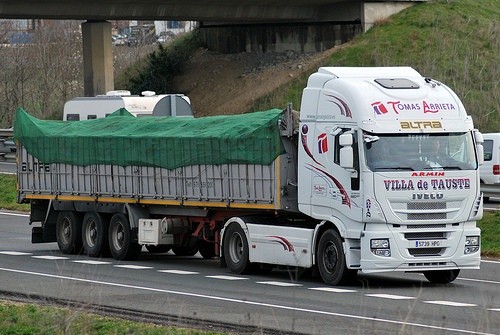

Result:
[63,90,190,121]
[480,132,500,205]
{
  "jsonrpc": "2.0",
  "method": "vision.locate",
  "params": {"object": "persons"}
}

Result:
[425,138,454,169]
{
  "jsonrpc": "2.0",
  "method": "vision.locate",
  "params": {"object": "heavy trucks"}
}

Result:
[11,63,482,288]
